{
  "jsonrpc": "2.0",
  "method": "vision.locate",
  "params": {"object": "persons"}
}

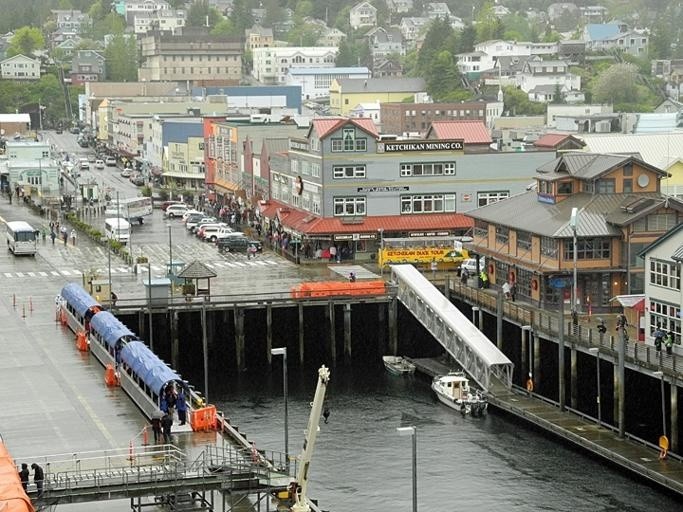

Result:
[18,463,44,491]
[142,176,354,263]
[67,300,187,447]
[501,281,674,358]
[87,276,118,305]
[349,241,490,290]
[0,175,111,246]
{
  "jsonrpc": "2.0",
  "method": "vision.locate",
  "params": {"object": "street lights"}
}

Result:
[164,222,173,304]
[467,304,669,457]
[393,422,419,511]
[268,345,292,478]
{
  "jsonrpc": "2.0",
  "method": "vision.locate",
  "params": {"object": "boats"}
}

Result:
[382,354,416,377]
[429,366,489,416]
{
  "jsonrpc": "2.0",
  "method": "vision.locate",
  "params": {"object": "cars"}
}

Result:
[121,159,264,255]
[62,123,118,173]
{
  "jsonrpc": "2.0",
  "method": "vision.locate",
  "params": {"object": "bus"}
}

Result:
[103,196,153,225]
[104,217,132,247]
[5,220,39,258]
[376,234,474,274]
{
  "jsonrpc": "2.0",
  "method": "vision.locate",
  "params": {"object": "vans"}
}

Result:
[457,257,477,277]
